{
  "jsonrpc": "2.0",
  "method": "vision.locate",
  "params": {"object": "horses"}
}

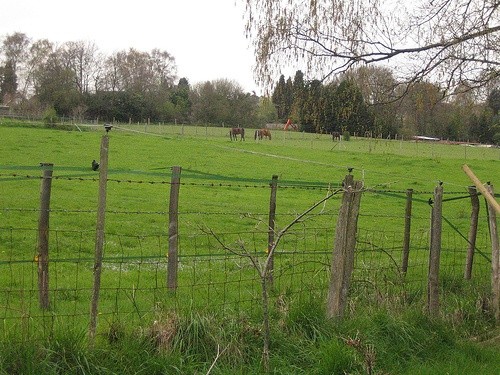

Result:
[230,128,245,142]
[332,132,340,140]
[255,129,272,141]
